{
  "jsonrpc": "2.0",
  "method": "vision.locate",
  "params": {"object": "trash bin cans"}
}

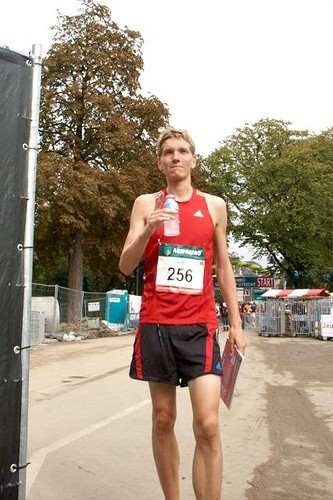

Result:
[104,289,129,325]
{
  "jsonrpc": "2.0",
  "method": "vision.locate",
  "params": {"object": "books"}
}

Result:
[220,335,244,409]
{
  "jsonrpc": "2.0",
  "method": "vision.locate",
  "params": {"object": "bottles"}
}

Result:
[163,195,180,236]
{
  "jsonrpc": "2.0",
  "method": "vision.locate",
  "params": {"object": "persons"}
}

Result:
[119,127,248,500]
[214,296,305,332]
